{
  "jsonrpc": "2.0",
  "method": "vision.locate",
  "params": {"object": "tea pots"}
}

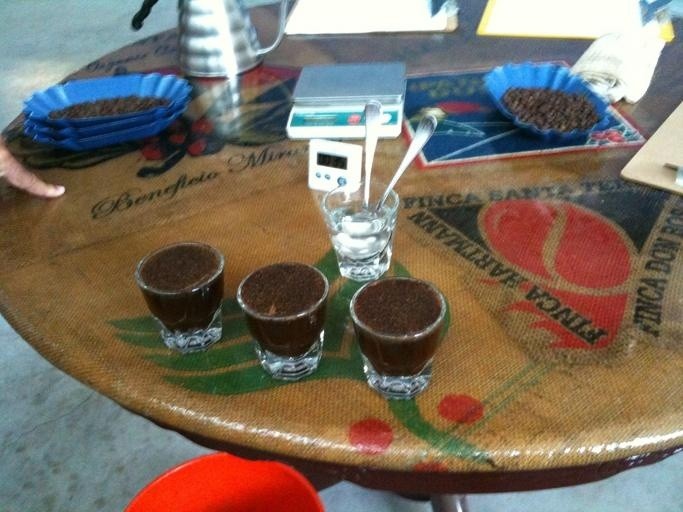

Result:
[133,0,288,78]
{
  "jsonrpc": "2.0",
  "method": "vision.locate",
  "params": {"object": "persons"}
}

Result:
[1,134,66,198]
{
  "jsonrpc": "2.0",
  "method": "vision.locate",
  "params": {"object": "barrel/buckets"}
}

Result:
[124,452,324,512]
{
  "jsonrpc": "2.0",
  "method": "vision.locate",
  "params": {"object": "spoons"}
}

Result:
[341,98,437,262]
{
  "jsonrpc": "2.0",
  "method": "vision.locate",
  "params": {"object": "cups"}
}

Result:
[320,182,401,280]
[135,242,226,354]
[348,276,447,396]
[235,264,330,379]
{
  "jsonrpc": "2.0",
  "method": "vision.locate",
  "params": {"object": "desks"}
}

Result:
[0,2,683,512]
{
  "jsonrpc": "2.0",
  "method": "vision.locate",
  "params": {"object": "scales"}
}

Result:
[286,63,407,139]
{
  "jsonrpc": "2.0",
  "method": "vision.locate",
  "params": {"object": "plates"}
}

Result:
[484,59,611,140]
[23,73,193,153]
